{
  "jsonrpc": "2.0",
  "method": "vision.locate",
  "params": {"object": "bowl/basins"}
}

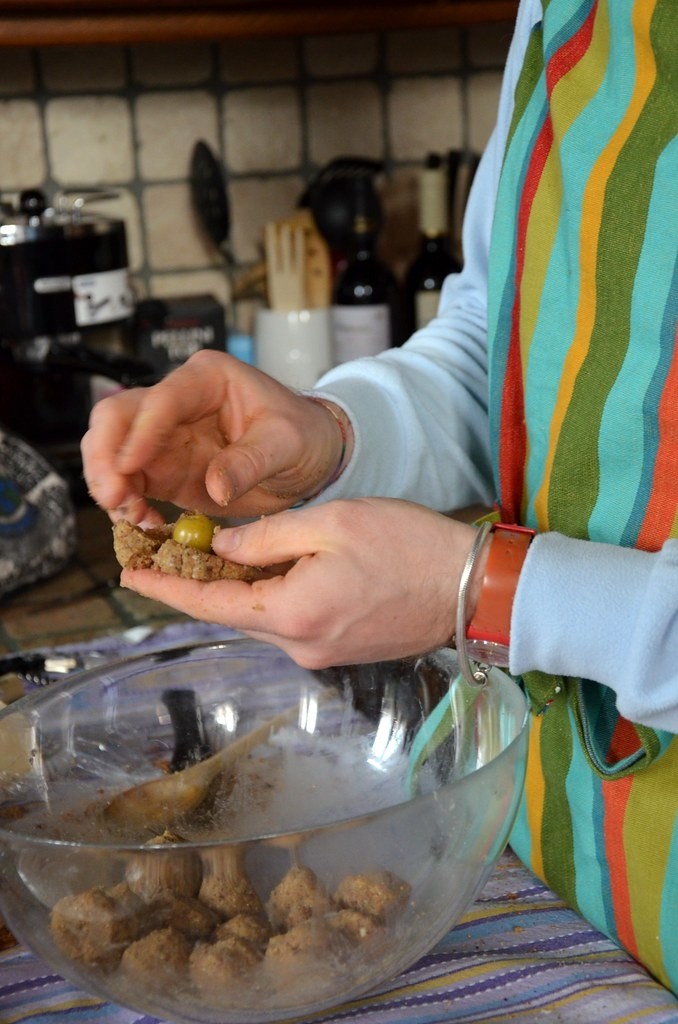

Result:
[0,637,530,1024]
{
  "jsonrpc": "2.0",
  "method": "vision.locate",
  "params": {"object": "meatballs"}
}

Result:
[57,850,409,1016]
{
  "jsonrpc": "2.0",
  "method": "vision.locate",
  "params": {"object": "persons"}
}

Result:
[80,1,677,998]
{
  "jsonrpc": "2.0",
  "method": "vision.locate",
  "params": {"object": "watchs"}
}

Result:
[466,524,536,668]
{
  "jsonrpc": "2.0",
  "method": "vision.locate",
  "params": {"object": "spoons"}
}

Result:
[101,688,339,841]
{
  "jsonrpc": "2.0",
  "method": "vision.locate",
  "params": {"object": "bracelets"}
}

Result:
[289,396,346,509]
[455,522,492,689]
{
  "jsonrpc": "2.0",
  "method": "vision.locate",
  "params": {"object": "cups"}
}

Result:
[256,308,334,394]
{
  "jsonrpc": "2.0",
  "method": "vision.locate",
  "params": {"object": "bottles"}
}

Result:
[402,153,462,351]
[332,198,403,365]
